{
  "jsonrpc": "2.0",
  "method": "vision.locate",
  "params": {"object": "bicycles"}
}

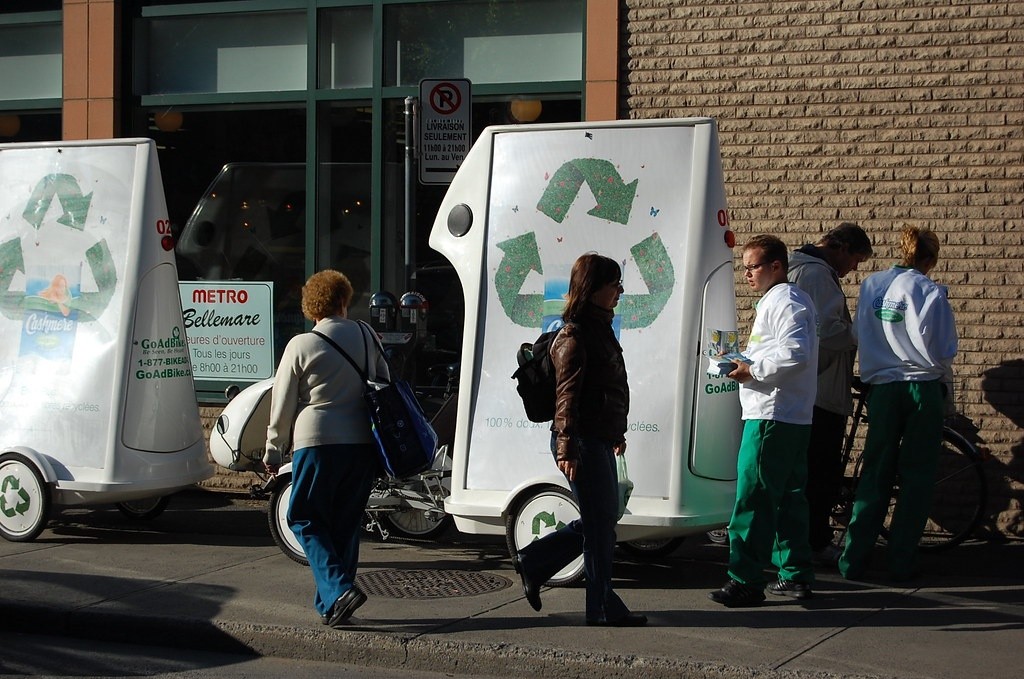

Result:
[829,375,989,549]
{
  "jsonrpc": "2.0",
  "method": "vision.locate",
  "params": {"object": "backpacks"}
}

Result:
[511,323,592,422]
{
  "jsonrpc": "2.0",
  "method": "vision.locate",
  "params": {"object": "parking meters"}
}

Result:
[370,292,429,394]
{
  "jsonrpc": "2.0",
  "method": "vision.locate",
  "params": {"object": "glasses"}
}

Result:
[743,262,771,271]
[604,280,623,287]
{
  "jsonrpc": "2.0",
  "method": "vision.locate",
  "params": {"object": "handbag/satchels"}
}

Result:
[368,382,437,480]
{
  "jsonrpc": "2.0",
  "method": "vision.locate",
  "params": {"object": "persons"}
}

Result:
[837,224,958,581]
[707,233,820,604]
[265,271,391,627]
[512,253,650,626]
[783,222,875,561]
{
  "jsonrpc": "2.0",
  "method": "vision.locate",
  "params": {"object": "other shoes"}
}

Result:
[812,544,839,568]
[322,586,366,627]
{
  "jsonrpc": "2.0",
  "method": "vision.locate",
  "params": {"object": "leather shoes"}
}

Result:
[512,543,547,612]
[586,611,647,626]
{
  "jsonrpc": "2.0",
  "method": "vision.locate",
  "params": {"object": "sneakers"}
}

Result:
[768,579,812,598]
[707,579,766,605]
